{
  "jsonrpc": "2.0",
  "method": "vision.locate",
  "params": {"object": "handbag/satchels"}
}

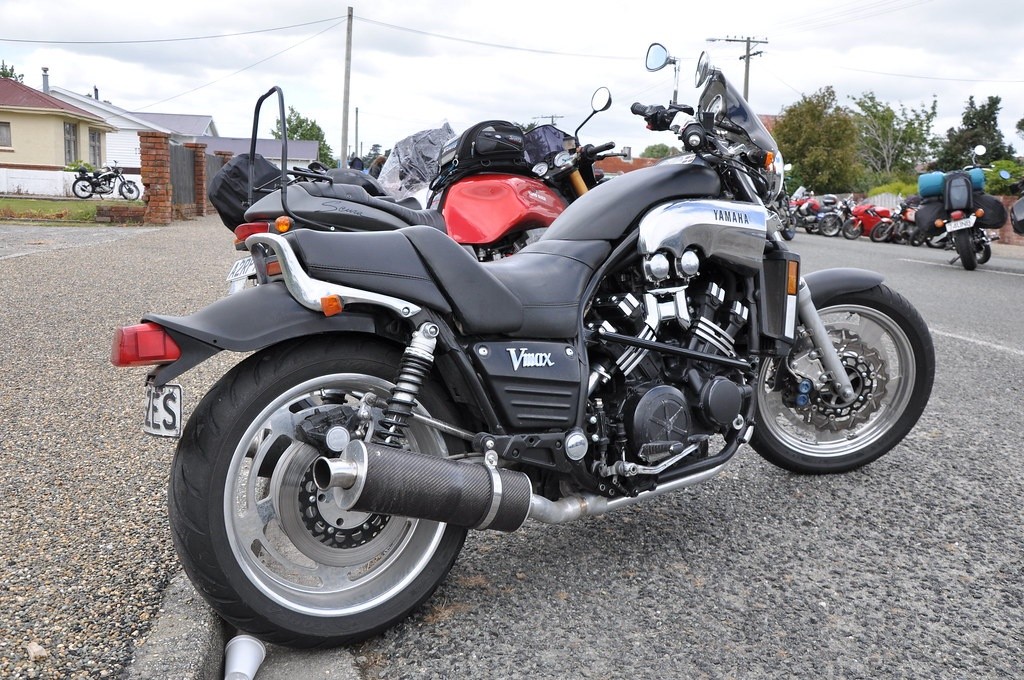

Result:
[429,120,526,192]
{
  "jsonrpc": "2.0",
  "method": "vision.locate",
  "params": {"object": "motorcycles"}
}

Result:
[782,184,955,249]
[109,42,936,651]
[72,160,140,202]
[916,145,1010,270]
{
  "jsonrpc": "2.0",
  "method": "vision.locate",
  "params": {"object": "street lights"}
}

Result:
[706,38,750,103]
